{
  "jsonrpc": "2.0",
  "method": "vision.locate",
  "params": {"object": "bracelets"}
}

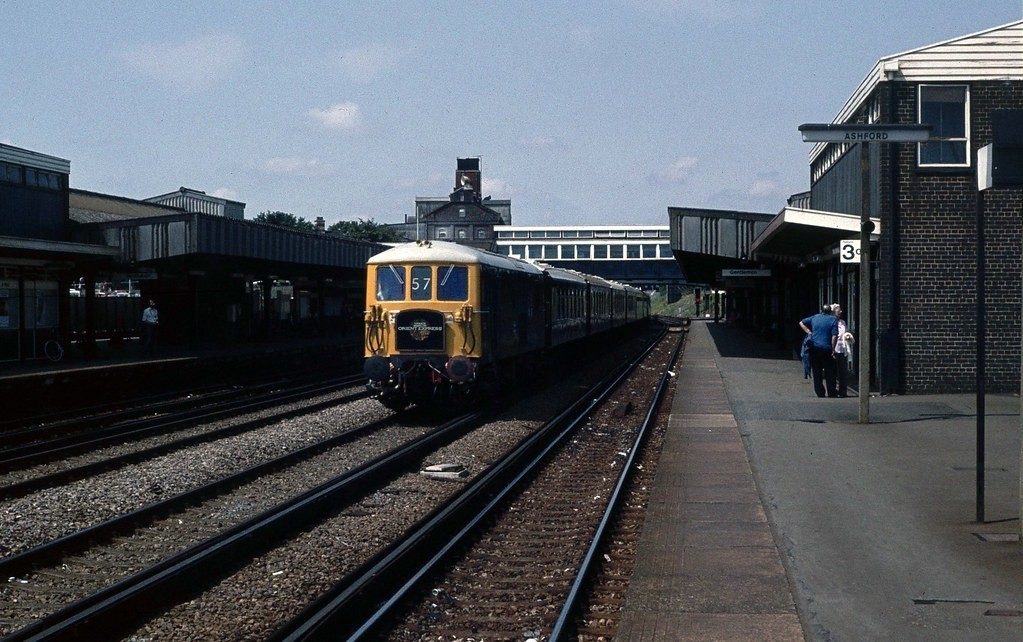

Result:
[806,329,810,334]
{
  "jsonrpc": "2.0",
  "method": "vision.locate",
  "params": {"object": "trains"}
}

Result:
[364,239,652,412]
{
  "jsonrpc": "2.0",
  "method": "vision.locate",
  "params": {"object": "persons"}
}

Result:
[829,303,850,397]
[142,299,160,360]
[799,304,839,397]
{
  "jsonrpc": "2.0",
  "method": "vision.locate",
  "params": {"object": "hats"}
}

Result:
[829,304,840,310]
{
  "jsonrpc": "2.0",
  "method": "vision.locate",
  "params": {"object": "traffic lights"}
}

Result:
[695,288,701,303]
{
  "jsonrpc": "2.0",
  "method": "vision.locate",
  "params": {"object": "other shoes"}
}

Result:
[828,395,838,398]
[836,389,840,395]
[839,394,848,398]
[818,394,826,398]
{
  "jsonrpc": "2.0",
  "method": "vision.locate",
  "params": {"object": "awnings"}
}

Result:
[750,206,881,258]
